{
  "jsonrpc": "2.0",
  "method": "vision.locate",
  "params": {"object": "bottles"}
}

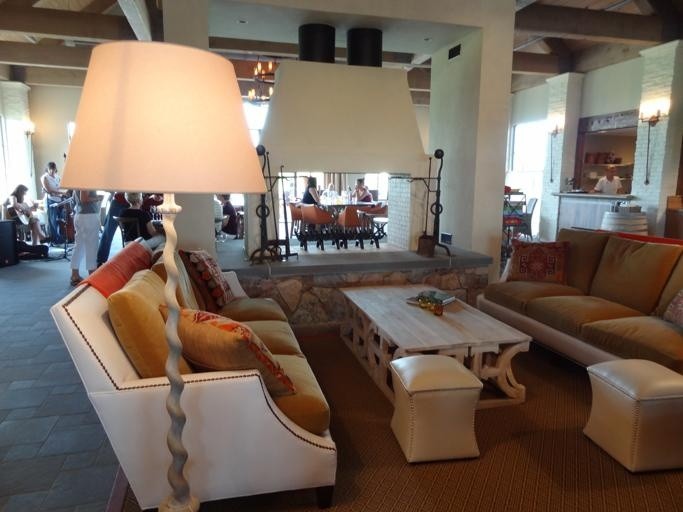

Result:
[433,300,444,317]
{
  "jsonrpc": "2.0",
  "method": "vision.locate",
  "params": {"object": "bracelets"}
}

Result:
[356,191,358,192]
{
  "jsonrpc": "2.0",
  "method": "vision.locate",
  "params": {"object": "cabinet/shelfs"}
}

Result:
[504,192,527,232]
[582,162,634,182]
[664,208,683,241]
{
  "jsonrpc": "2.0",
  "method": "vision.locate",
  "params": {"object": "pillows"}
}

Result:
[106,249,296,397]
[506,227,683,328]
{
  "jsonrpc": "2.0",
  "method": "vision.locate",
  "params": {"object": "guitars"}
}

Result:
[9,202,38,225]
[57,195,75,243]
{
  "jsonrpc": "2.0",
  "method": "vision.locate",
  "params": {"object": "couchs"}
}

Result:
[476,226,683,376]
[50,234,337,512]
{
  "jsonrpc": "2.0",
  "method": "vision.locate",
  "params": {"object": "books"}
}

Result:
[405,291,456,307]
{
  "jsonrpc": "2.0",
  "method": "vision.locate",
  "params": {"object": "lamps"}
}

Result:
[58,41,269,512]
[638,95,671,186]
[253,60,275,85]
[257,23,428,176]
[545,113,563,183]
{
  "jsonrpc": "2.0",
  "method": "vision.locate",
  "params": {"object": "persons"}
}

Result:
[220,194,237,235]
[587,164,625,194]
[3,184,50,246]
[352,179,372,218]
[119,191,165,250]
[70,189,104,286]
[41,162,69,246]
[97,191,164,268]
[300,178,320,233]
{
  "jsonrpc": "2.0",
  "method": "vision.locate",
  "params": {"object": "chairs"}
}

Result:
[2,205,26,242]
[288,199,388,249]
[112,215,142,247]
[514,198,538,241]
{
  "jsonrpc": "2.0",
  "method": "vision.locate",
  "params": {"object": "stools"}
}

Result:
[583,359,683,476]
[505,218,521,259]
[388,354,483,463]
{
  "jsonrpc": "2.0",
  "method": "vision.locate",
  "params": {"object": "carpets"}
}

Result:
[106,325,683,507]
